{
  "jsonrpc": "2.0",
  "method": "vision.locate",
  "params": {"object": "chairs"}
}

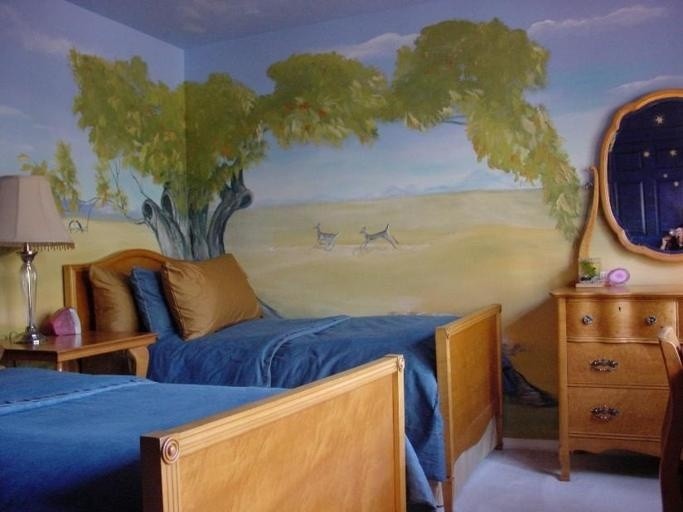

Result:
[655,326,683,511]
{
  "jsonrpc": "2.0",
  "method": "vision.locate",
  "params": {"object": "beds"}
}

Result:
[0,352,406,510]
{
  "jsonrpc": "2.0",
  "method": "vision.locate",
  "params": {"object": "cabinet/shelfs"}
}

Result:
[554,295,682,482]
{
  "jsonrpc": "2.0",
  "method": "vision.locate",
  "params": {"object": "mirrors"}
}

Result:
[598,89,683,264]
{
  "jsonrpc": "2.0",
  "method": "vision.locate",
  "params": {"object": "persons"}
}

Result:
[659,226,683,250]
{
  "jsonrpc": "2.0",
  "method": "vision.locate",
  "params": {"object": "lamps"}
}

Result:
[0,174,78,344]
